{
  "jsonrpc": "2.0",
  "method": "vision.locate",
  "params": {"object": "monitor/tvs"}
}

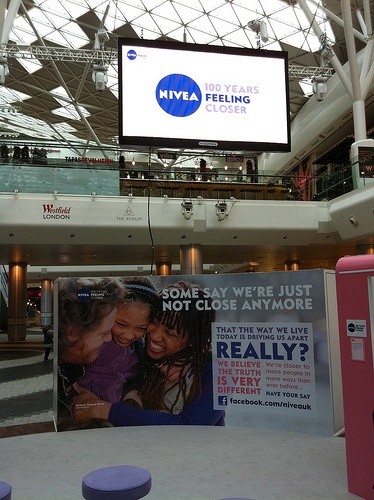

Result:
[117,37,291,153]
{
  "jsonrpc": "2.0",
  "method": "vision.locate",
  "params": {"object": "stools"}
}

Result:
[82,465,152,500]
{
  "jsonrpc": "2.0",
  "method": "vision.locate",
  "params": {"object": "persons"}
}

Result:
[71,280,226,427]
[57,277,143,432]
[79,277,159,404]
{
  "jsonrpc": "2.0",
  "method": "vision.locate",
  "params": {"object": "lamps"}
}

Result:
[91,64,109,93]
[313,76,327,102]
